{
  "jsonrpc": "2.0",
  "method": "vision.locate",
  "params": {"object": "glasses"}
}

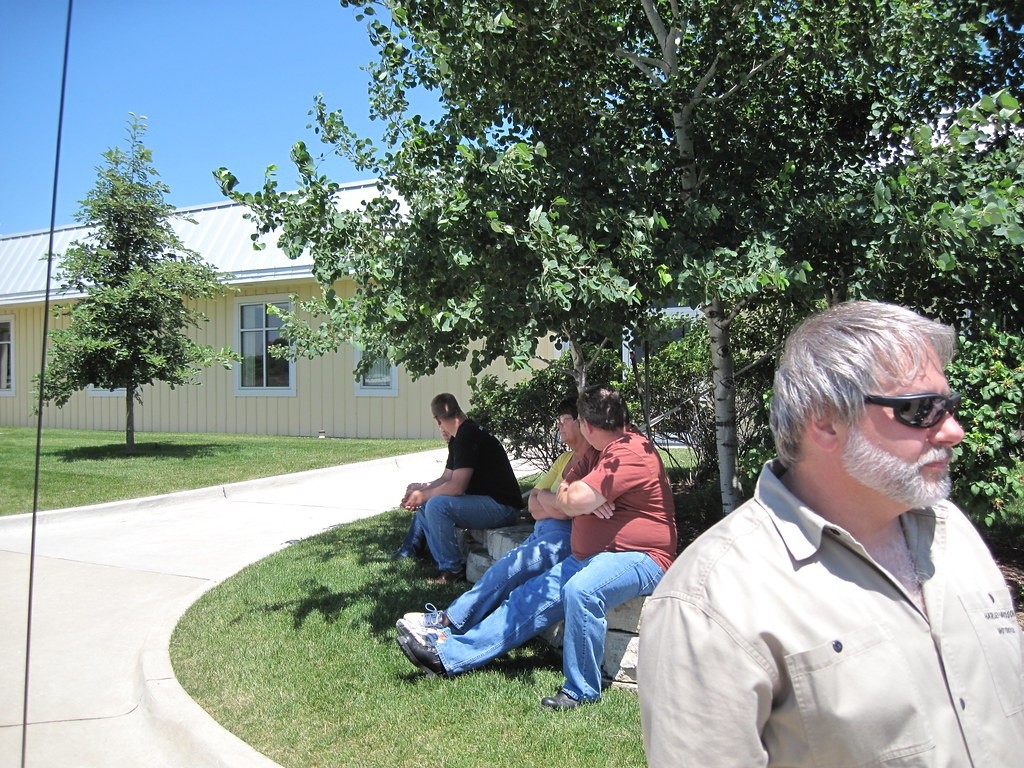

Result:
[865,394,962,429]
[555,416,577,424]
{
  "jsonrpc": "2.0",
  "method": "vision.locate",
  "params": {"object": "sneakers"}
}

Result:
[403,602,444,628]
[396,618,448,646]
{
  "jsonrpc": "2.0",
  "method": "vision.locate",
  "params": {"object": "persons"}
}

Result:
[395,386,679,708]
[637,300,1024,768]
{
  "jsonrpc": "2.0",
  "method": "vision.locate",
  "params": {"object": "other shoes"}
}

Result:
[439,566,466,583]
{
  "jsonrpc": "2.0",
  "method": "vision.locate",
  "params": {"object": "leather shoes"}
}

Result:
[396,634,445,679]
[541,691,580,711]
